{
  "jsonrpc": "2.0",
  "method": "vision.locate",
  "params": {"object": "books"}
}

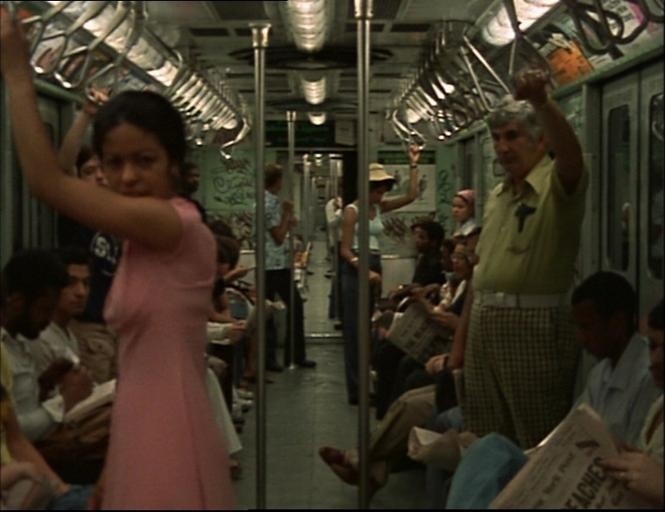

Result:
[68,381,116,421]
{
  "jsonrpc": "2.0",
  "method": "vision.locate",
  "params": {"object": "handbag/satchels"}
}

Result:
[3,477,53,511]
[434,356,459,413]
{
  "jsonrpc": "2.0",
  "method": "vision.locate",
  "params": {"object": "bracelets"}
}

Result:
[410,163,418,169]
[351,255,359,262]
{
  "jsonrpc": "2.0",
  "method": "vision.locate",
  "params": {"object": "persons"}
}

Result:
[452,69,589,452]
[39,250,119,380]
[0,246,119,486]
[54,83,126,324]
[374,239,458,372]
[452,189,478,238]
[338,143,420,405]
[189,159,315,481]
[367,244,471,411]
[323,193,342,323]
[372,222,445,323]
[0,5,237,511]
[446,297,665,511]
[319,226,482,487]
[1,382,104,511]
[373,270,665,508]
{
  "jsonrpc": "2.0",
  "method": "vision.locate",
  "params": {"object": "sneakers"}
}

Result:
[239,399,253,410]
[270,366,284,373]
[349,398,376,407]
[285,361,316,368]
[239,390,254,399]
[234,417,244,424]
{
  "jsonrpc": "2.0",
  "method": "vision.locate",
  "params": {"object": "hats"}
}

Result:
[456,189,475,203]
[368,162,396,183]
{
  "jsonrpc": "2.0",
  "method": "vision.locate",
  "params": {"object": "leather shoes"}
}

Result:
[318,446,354,467]
[331,464,379,498]
[231,465,245,481]
[244,374,273,383]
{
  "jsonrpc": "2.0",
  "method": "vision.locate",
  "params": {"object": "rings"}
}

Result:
[621,473,628,483]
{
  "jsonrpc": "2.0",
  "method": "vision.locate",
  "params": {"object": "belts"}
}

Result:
[472,290,569,310]
